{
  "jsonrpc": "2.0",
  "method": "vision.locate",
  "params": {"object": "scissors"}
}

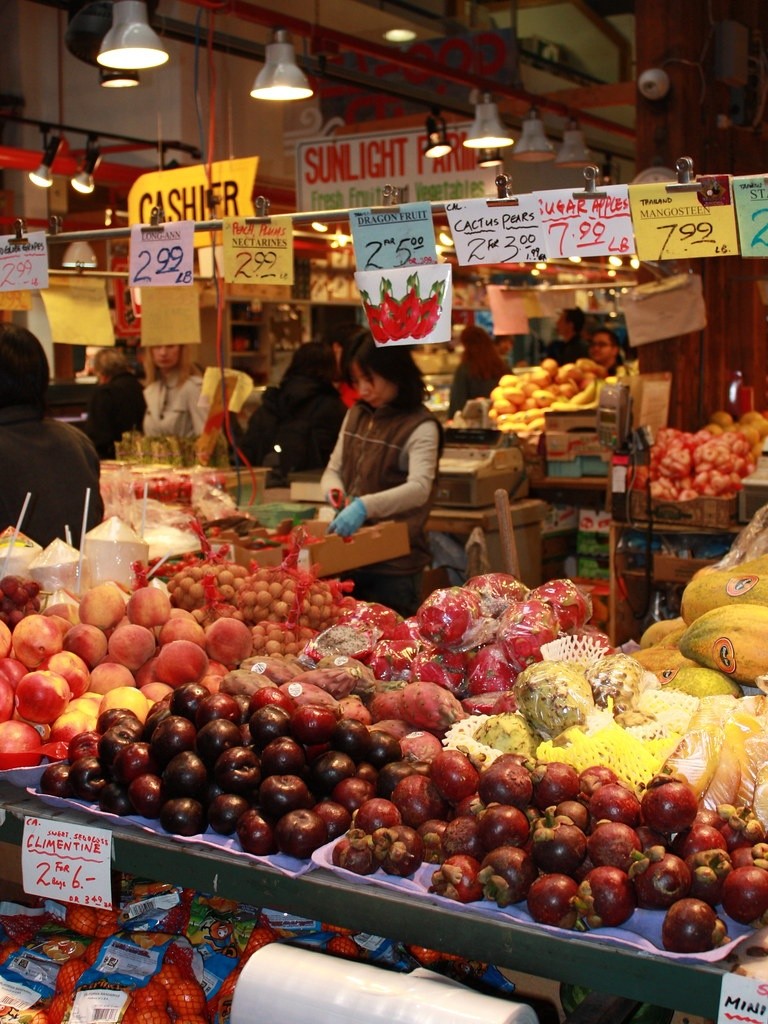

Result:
[328,488,351,520]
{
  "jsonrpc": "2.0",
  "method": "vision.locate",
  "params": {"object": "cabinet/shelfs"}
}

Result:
[607,519,747,650]
[226,301,272,384]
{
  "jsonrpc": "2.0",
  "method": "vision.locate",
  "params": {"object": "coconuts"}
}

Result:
[0,515,149,605]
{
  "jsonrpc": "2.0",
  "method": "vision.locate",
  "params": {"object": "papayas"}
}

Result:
[627,549,768,705]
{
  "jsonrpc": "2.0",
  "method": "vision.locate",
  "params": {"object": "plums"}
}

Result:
[38,683,406,862]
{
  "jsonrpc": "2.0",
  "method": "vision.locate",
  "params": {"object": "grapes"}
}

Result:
[0,575,41,628]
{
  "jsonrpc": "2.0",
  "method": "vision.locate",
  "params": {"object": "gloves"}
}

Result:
[329,497,367,536]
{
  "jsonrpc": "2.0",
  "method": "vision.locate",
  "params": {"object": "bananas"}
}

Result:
[572,377,607,408]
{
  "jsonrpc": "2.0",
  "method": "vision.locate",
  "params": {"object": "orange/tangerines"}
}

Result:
[0,882,469,1024]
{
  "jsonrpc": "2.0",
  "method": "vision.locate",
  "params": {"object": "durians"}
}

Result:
[701,408,768,453]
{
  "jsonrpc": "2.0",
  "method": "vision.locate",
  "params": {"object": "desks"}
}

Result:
[258,485,547,594]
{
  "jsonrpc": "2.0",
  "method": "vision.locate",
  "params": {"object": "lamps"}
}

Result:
[28,126,61,188]
[251,28,314,102]
[514,102,558,163]
[70,133,102,195]
[423,106,451,159]
[463,89,514,168]
[555,114,595,168]
[97,0,170,88]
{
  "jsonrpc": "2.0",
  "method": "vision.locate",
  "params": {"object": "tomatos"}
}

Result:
[148,552,199,577]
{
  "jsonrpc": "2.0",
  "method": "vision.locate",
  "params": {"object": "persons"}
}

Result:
[318,327,448,619]
[0,325,104,552]
[80,307,621,482]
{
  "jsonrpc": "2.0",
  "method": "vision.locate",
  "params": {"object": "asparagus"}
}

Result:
[114,431,229,468]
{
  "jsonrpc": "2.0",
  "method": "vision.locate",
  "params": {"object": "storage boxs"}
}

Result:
[541,501,612,625]
[547,409,613,477]
[222,466,317,530]
[652,551,721,582]
[206,519,410,578]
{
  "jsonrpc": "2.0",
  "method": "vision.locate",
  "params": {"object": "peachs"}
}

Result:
[0,584,253,771]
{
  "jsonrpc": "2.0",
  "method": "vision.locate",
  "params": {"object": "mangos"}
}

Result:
[489,358,609,432]
